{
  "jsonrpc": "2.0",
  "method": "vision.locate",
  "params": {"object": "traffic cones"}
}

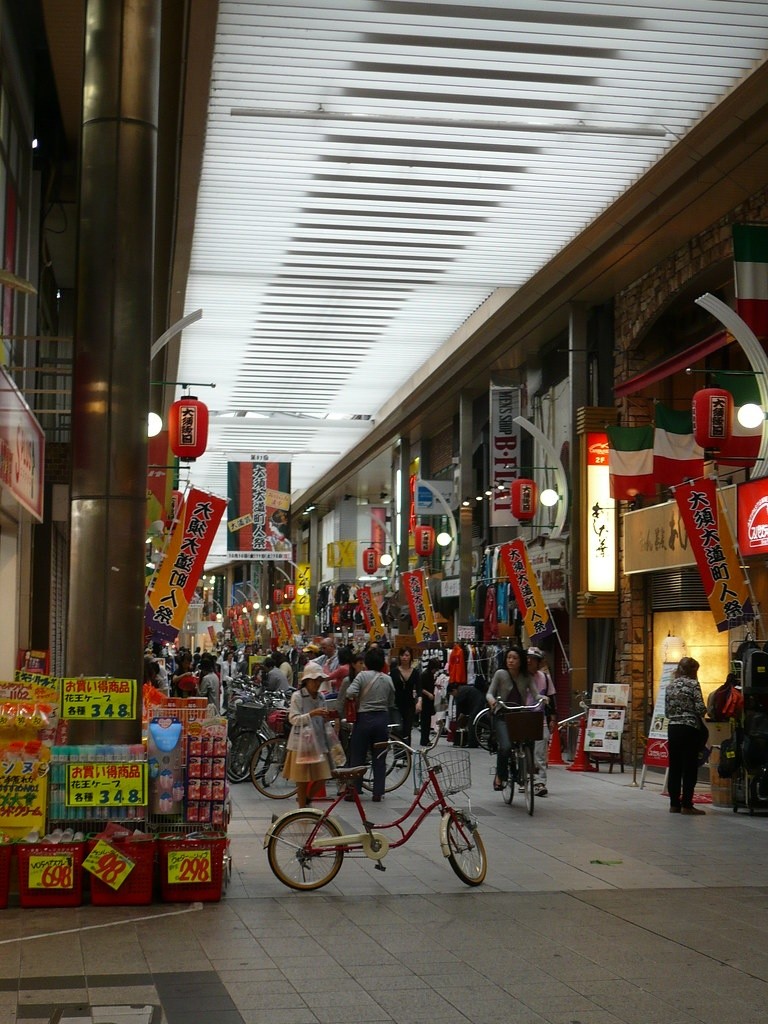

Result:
[548,722,569,765]
[566,717,599,772]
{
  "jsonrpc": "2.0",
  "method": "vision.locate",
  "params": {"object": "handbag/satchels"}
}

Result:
[296,714,326,764]
[324,720,347,767]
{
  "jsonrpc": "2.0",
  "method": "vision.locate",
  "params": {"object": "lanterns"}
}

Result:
[661,636,688,663]
[692,389,734,453]
[167,396,208,462]
[512,476,538,522]
[415,524,435,557]
[274,584,295,604]
[211,613,216,621]
[363,548,377,574]
[228,600,252,618]
[162,487,184,529]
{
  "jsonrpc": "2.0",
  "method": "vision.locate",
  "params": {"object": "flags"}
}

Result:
[714,373,763,467]
[606,424,656,500]
[653,401,703,486]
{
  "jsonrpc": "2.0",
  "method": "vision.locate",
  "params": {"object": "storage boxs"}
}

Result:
[160,832,227,901]
[15,840,84,906]
[0,842,15,908]
[88,835,157,904]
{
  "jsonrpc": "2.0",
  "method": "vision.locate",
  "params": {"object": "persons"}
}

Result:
[345,649,396,803]
[419,659,440,745]
[389,647,422,767]
[143,638,364,715]
[283,662,329,808]
[337,653,366,796]
[664,657,707,814]
[486,647,556,796]
[446,682,486,721]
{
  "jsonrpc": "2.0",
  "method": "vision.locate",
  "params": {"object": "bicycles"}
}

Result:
[491,695,550,816]
[226,671,413,799]
[262,720,488,892]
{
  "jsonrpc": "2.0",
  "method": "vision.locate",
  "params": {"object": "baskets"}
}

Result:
[413,750,471,798]
[236,703,265,727]
[506,711,544,743]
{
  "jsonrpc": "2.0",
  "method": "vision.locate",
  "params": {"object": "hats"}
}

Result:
[527,646,543,658]
[301,661,329,680]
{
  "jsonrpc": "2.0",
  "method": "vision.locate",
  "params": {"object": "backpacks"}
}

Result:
[717,640,768,808]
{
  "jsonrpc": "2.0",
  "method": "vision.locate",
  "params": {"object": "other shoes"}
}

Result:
[344,795,354,802]
[492,773,503,790]
[518,784,526,793]
[463,744,479,748]
[670,807,681,813]
[533,784,547,796]
[427,742,433,746]
[680,806,705,815]
[373,795,381,802]
